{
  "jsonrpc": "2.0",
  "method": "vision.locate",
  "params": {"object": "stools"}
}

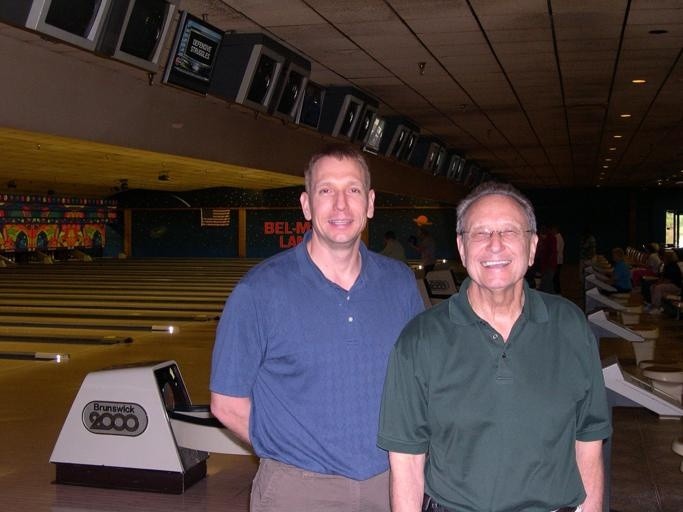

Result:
[639,359,683,414]
[671,302,682,318]
[667,294,681,313]
[626,323,660,366]
[611,292,630,308]
[642,366,683,421]
[620,305,643,325]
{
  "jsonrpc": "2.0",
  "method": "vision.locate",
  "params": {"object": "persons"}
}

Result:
[209,147,425,512]
[526,226,566,294]
[379,228,434,260]
[581,231,683,314]
[376,180,613,512]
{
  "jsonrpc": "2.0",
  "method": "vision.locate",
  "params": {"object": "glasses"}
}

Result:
[461,229,531,240]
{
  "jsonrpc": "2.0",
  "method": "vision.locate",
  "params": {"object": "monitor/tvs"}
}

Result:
[432,146,449,176]
[406,136,427,169]
[350,104,378,147]
[210,34,285,115]
[0,0,114,53]
[93,0,181,75]
[161,10,225,99]
[321,86,364,140]
[293,79,326,130]
[421,138,441,170]
[360,112,386,157]
[271,62,311,122]
[398,128,420,165]
[444,150,462,179]
[382,123,410,159]
[453,157,467,182]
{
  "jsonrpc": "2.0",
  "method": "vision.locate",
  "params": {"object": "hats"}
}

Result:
[651,242,659,250]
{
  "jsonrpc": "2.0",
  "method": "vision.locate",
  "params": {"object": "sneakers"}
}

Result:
[649,307,664,315]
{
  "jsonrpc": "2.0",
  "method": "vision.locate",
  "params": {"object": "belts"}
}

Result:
[422,491,578,512]
